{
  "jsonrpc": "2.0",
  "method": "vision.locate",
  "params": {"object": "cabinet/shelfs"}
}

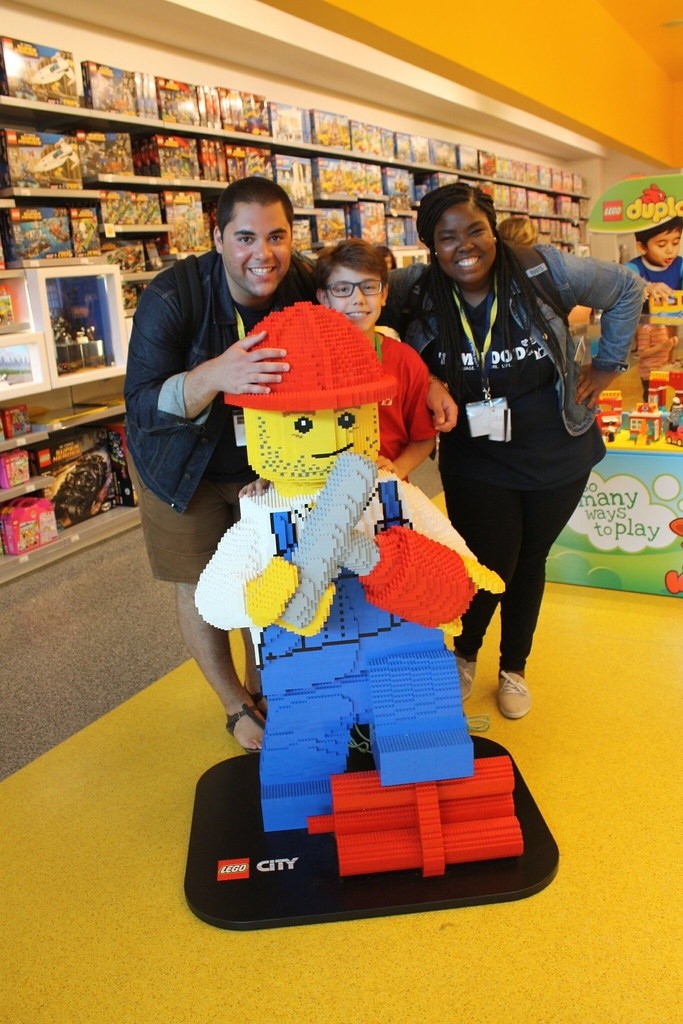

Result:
[0,94,592,585]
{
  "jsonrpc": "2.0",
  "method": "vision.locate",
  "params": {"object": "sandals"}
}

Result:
[224,683,267,753]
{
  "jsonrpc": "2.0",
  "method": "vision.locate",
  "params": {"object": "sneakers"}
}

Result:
[498,667,531,719]
[454,651,477,702]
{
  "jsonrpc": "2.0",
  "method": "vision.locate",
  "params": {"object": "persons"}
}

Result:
[372,182,649,722]
[239,239,438,498]
[124,176,460,755]
[638,318,678,403]
[197,302,507,833]
[625,215,683,304]
[497,218,535,246]
[377,246,398,271]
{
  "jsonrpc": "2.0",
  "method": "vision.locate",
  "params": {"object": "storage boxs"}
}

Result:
[0,35,588,559]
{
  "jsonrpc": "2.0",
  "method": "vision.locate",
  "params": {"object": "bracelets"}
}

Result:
[429,376,449,391]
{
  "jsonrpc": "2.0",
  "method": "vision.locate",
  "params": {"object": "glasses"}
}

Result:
[325,278,385,298]
